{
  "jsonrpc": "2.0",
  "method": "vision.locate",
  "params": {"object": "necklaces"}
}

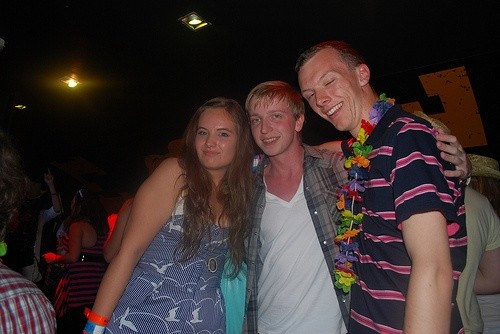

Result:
[334,93,395,293]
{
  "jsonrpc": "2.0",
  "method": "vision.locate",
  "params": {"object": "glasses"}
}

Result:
[77,189,83,198]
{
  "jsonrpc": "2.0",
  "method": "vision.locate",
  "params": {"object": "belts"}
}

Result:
[79,254,105,262]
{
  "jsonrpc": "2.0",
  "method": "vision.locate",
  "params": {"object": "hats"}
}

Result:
[467,154,500,178]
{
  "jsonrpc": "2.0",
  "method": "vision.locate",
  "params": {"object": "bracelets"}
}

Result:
[84,321,105,334]
[83,308,108,326]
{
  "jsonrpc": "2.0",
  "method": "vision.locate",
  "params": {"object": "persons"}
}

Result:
[4,168,62,282]
[104,153,168,264]
[84,98,254,334]
[239,81,472,334]
[41,189,108,334]
[457,188,500,334]
[296,41,464,334]
[0,127,58,334]
